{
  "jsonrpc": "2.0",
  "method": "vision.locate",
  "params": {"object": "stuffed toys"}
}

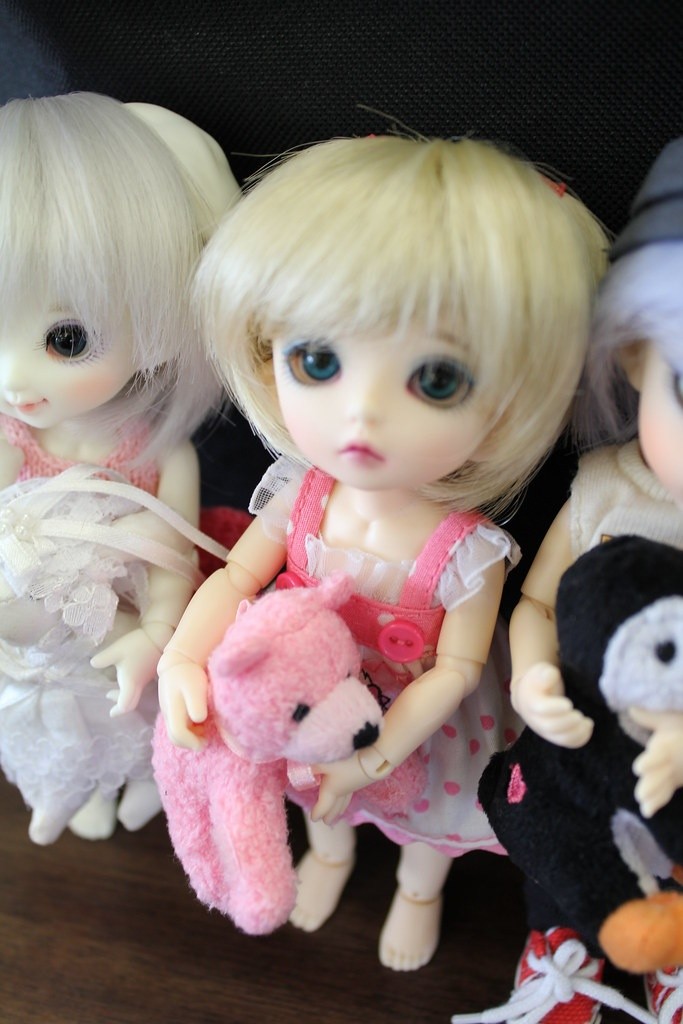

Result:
[151,575,383,935]
[479,534,683,1023]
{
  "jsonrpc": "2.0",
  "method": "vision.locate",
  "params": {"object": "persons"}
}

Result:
[0,92,683,1024]
